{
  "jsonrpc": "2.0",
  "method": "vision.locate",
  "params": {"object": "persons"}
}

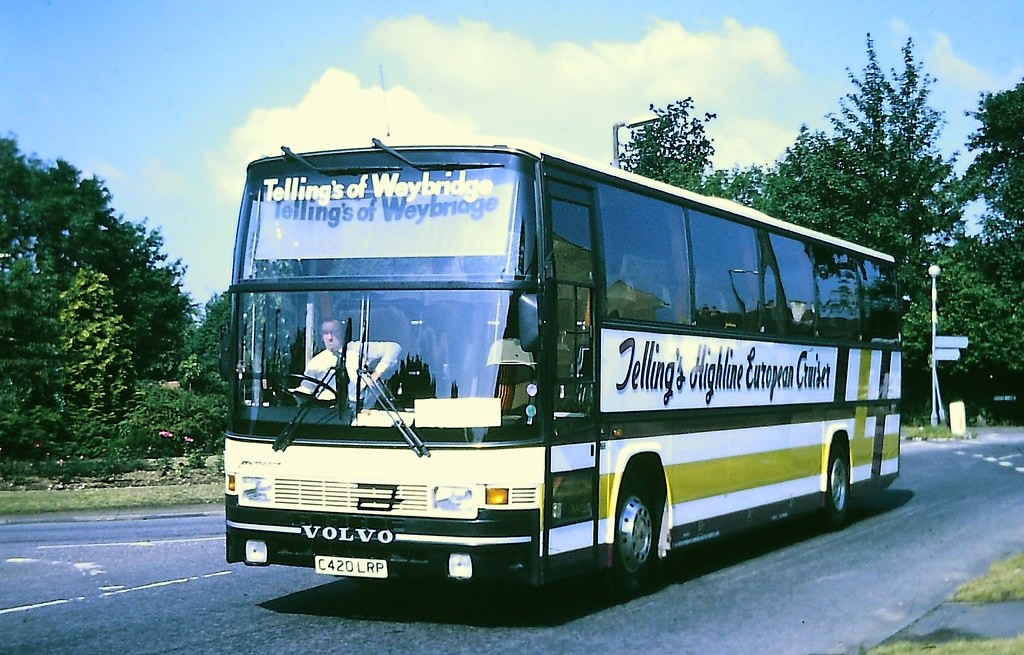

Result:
[287,318,402,404]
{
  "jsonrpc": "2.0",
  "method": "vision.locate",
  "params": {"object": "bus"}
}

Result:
[221,137,902,596]
